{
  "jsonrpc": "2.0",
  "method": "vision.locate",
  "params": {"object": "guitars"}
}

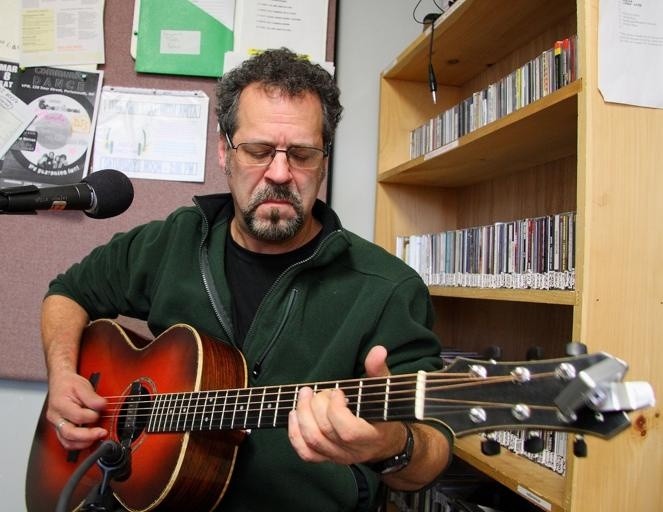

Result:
[27,319,655,512]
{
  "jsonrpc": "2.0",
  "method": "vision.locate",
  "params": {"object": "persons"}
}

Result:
[37,150,68,171]
[39,47,454,511]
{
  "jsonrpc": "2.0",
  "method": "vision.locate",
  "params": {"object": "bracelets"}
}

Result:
[366,416,413,476]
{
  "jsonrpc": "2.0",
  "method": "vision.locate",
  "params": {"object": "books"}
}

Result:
[439,347,568,477]
[396,210,576,291]
[410,35,575,160]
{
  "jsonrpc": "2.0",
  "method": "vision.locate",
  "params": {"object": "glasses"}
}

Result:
[225,132,328,170]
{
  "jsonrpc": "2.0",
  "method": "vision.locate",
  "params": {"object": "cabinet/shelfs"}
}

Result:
[372,1,663,512]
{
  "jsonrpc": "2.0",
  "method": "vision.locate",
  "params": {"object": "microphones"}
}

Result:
[0,169,134,219]
